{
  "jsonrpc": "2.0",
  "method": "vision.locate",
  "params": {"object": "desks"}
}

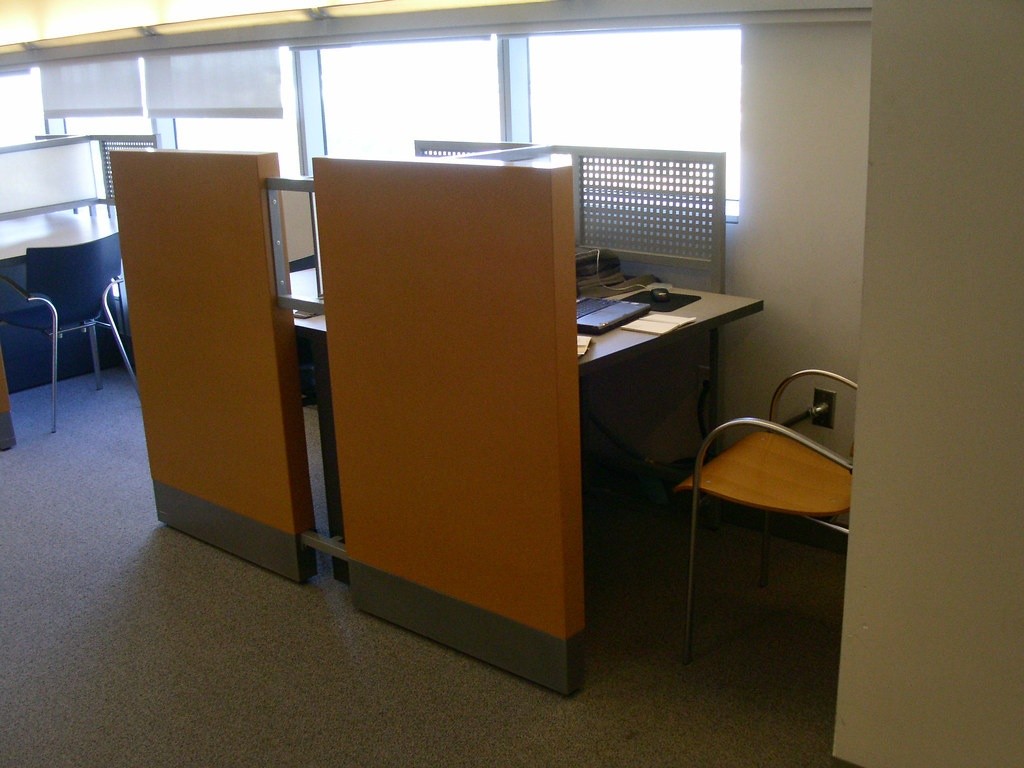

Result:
[577,283,766,528]
[285,264,348,579]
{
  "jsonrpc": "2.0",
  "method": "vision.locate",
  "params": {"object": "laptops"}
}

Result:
[576,297,651,333]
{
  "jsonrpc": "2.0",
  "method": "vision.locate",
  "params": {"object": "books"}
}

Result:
[621,314,696,336]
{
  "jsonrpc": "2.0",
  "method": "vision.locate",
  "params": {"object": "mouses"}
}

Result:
[651,288,669,302]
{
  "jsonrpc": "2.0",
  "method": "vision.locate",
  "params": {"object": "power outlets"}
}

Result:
[813,386,837,428]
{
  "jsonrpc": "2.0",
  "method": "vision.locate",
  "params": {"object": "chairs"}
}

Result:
[672,367,861,665]
[0,229,140,437]
